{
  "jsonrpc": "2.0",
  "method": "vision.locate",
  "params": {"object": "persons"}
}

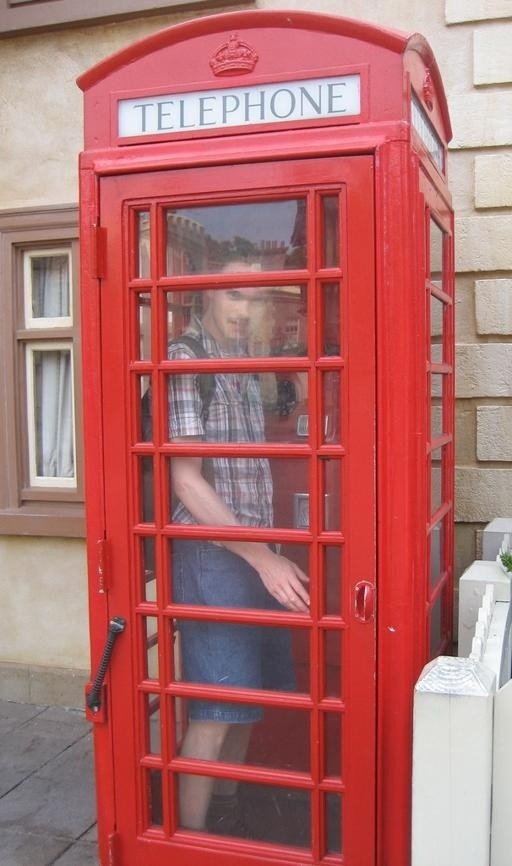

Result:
[149,246,314,835]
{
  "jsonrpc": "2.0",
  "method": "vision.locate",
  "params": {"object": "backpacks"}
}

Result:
[140,335,215,571]
